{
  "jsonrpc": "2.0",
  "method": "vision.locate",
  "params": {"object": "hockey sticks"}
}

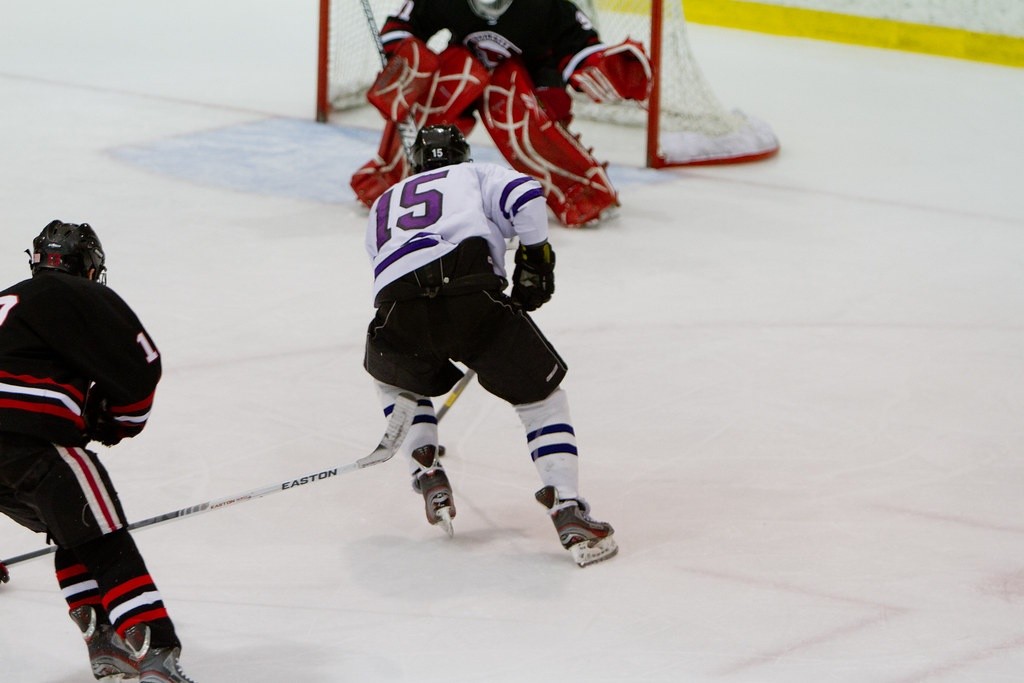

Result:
[359,0,420,156]
[32,389,420,560]
[435,276,537,421]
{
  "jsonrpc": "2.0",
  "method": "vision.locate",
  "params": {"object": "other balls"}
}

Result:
[438,445,445,456]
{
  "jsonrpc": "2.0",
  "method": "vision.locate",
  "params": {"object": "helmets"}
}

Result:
[410,122,471,169]
[33,219,109,285]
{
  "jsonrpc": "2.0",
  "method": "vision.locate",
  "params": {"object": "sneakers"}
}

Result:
[413,444,456,535]
[72,605,140,683]
[126,626,195,683]
[536,485,618,567]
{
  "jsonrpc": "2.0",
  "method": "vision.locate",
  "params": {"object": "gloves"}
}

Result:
[82,388,124,449]
[509,240,555,311]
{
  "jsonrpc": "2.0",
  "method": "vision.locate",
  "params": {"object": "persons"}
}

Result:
[363,124,619,568]
[0,219,194,683]
[350,0,655,228]
